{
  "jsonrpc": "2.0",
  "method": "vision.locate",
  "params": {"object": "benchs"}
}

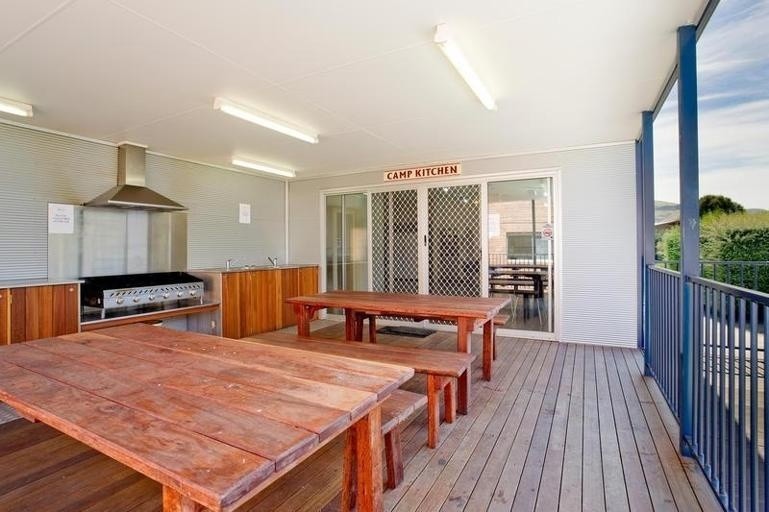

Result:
[380,389,428,489]
[362,314,509,360]
[243,332,477,449]
[488,279,548,323]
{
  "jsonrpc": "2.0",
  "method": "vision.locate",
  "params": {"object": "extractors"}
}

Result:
[80,143,190,212]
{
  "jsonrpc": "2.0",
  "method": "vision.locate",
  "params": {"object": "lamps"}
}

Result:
[213,97,319,144]
[231,155,295,178]
[0,98,33,118]
[434,23,497,112]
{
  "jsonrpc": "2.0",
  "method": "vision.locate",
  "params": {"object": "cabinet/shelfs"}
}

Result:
[276,266,319,329]
[9,283,80,344]
[0,287,9,345]
[187,269,276,338]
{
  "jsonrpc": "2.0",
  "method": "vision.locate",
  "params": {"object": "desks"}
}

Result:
[285,290,511,415]
[488,263,548,317]
[0,322,415,512]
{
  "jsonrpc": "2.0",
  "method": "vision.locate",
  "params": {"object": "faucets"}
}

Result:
[268,256,278,266]
[225,258,241,270]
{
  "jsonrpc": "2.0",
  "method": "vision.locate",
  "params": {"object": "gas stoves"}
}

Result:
[77,271,204,319]
